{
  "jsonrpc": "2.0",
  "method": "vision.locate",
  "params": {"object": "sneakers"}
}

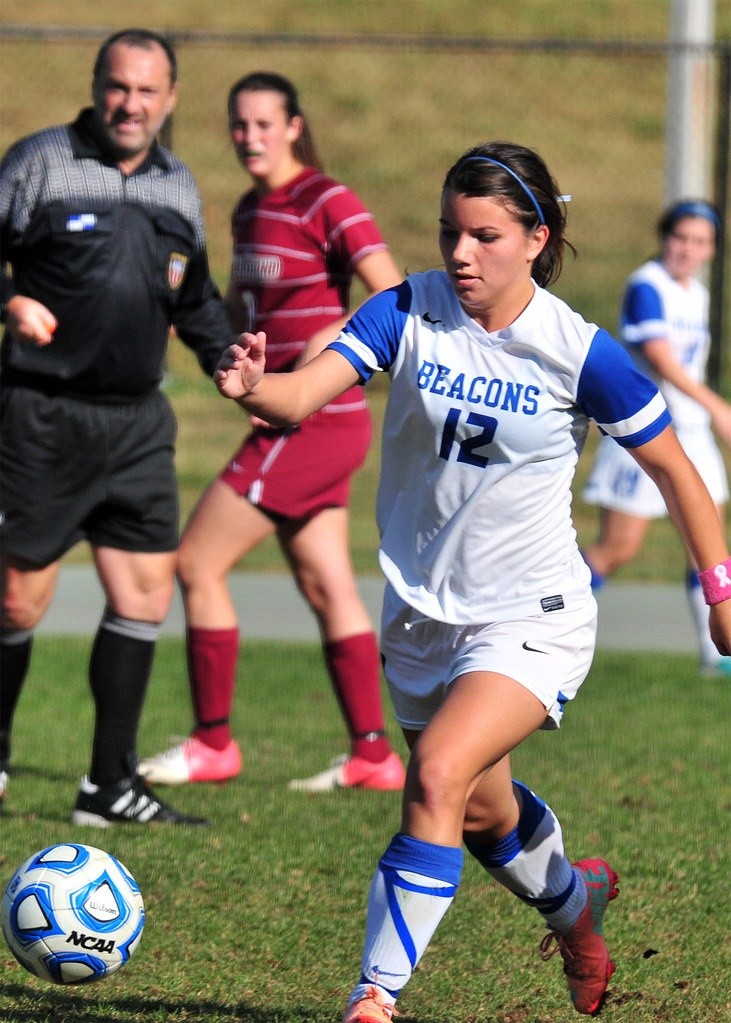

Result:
[343,971,399,1023]
[73,773,209,829]
[135,737,243,784]
[540,859,620,1014]
[288,751,406,791]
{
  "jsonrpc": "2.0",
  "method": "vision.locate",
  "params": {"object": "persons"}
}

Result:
[575,198,731,682]
[215,140,731,1023]
[0,27,242,827]
[135,72,406,791]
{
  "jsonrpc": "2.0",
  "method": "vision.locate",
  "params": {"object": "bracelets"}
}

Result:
[698,557,731,606]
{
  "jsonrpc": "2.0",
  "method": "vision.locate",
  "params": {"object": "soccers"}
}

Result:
[0,842,147,985]
